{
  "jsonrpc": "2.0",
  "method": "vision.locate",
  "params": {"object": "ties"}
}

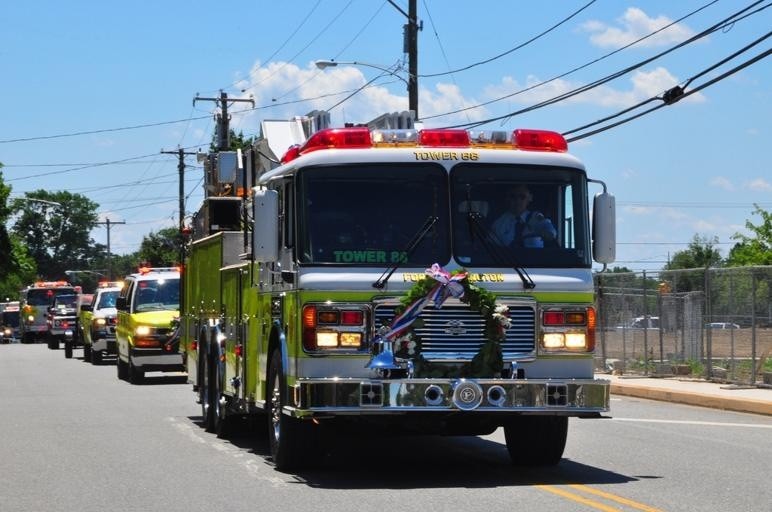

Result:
[514,216,523,248]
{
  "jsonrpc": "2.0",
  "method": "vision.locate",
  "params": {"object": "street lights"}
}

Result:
[316,59,419,121]
[7,196,62,208]
[63,270,105,280]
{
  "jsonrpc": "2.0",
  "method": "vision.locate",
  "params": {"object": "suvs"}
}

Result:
[46,294,78,349]
[64,293,93,358]
[616,315,659,330]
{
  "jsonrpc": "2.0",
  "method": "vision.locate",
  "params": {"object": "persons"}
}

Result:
[490,180,557,248]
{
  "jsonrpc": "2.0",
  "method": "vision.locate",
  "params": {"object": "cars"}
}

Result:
[704,322,740,329]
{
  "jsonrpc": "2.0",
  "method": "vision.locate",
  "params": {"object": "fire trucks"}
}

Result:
[178,108,615,471]
[0,297,21,341]
[114,266,186,385]
[79,282,127,365]
[20,281,82,344]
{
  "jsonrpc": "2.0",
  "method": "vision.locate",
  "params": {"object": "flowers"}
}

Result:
[387,263,513,379]
